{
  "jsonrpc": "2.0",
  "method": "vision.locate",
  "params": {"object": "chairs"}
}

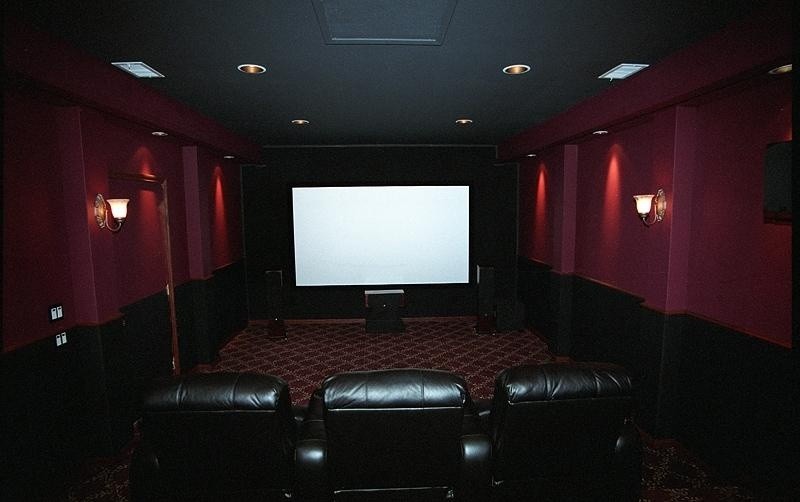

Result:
[126,364,298,501]
[294,369,494,500]
[473,359,648,501]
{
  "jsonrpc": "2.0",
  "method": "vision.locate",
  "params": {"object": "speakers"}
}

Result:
[266,269,286,337]
[477,264,496,333]
[364,289,406,309]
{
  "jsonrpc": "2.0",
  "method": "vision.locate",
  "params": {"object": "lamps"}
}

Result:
[629,189,669,227]
[93,189,131,233]
